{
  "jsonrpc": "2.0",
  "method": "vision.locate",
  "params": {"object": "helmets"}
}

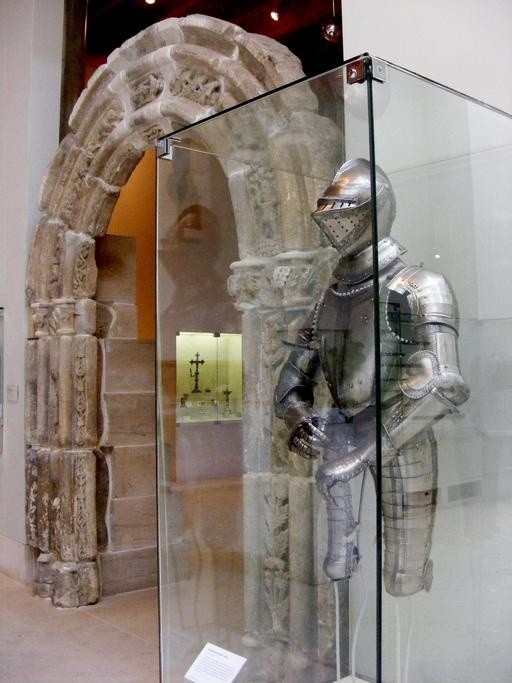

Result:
[311,156,397,259]
